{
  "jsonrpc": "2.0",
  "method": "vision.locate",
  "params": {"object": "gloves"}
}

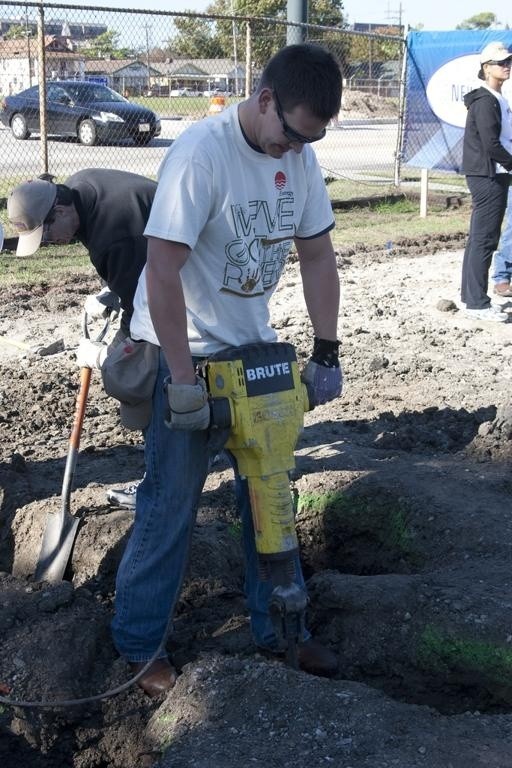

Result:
[75,339,106,371]
[163,373,211,432]
[84,287,120,322]
[302,336,342,406]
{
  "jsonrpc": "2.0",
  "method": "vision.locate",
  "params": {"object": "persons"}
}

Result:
[6,168,158,373]
[491,44,512,298]
[461,41,512,323]
[98,45,344,697]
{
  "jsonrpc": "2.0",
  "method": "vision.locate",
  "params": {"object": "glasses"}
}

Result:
[483,59,512,66]
[272,77,326,143]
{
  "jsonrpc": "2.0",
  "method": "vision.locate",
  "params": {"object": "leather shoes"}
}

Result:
[131,658,177,698]
[256,637,341,677]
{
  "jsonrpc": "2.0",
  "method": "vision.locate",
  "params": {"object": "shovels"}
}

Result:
[34,306,115,585]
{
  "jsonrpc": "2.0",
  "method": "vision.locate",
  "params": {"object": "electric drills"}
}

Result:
[161,342,317,670]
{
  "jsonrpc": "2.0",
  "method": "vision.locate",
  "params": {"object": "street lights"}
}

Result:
[225,8,242,96]
[142,23,152,90]
[21,0,31,90]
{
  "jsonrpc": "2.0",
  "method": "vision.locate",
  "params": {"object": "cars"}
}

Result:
[0,80,162,145]
[141,84,233,98]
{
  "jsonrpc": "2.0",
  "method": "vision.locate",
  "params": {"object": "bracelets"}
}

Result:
[311,337,342,368]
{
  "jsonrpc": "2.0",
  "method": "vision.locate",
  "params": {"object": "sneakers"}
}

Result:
[493,282,512,297]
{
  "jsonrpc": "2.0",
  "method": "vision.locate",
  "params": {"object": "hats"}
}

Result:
[480,41,512,64]
[101,337,160,431]
[7,178,58,258]
